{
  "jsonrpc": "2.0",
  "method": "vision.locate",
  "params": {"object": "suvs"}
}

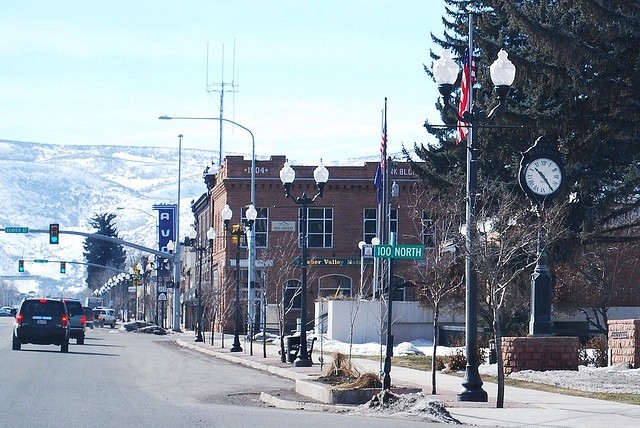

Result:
[11,297,70,353]
[63,296,87,345]
[82,307,94,330]
[92,308,116,328]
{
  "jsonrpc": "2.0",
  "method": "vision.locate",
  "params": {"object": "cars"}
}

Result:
[0,307,18,317]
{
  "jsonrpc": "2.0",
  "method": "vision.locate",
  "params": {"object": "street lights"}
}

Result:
[424,46,526,402]
[183,226,217,344]
[172,135,183,336]
[273,158,335,367]
[116,240,181,331]
[159,114,257,347]
[91,276,116,308]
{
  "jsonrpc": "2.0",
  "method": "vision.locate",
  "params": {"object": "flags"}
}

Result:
[375,126,388,201]
[454,44,477,147]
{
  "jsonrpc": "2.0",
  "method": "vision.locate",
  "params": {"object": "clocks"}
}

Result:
[524,157,563,196]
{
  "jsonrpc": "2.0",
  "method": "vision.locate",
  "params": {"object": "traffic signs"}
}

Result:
[5,227,28,233]
[374,244,426,260]
[34,259,48,263]
[217,202,261,353]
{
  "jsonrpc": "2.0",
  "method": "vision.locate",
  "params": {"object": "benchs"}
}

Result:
[278,337,317,364]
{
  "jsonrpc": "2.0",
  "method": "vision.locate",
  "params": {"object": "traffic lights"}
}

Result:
[19,260,24,272]
[49,224,59,244]
[61,262,65,273]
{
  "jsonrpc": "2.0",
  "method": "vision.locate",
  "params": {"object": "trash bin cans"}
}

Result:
[288,337,301,363]
